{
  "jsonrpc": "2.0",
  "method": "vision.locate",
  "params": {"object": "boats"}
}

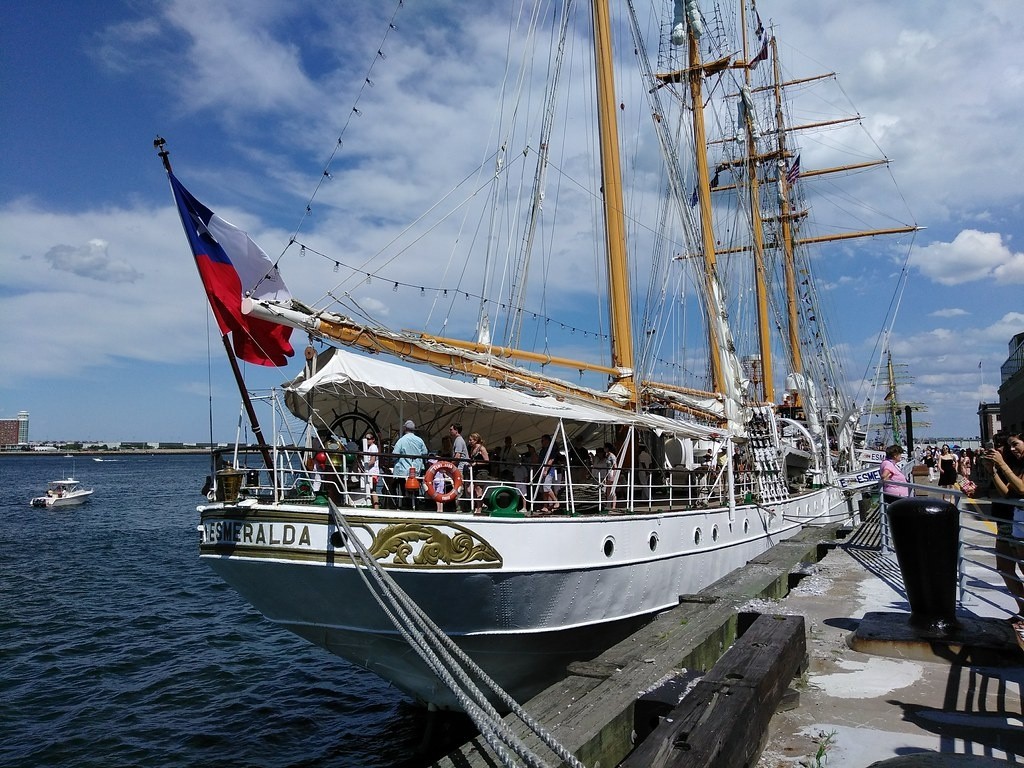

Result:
[29,460,95,506]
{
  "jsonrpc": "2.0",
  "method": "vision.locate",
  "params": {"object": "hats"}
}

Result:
[720,445,727,450]
[702,453,713,458]
[402,420,415,430]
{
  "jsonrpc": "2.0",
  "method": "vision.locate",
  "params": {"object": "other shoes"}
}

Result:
[930,478,932,482]
[474,504,488,515]
[541,507,548,512]
[552,504,559,512]
[1007,611,1023,624]
[518,507,527,513]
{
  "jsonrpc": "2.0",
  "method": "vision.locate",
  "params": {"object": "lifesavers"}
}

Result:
[425,460,462,502]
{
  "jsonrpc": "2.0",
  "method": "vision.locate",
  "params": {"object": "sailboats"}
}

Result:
[152,2,925,709]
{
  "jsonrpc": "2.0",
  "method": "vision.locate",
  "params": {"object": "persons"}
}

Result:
[568,435,591,470]
[365,433,380,509]
[589,448,607,485]
[536,434,561,515]
[919,451,935,483]
[880,444,914,499]
[980,430,1024,651]
[449,423,476,515]
[938,444,958,503]
[500,436,517,482]
[393,419,428,511]
[603,442,618,511]
[211,450,227,485]
[639,446,654,499]
[468,432,488,514]
[307,391,885,519]
[898,431,986,518]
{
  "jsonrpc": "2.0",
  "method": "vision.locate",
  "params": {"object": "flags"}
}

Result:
[168,171,294,367]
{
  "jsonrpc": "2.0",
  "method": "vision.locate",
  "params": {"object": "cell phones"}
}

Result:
[986,442,994,454]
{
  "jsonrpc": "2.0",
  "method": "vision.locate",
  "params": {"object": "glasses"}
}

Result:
[366,437,374,440]
[450,428,457,431]
